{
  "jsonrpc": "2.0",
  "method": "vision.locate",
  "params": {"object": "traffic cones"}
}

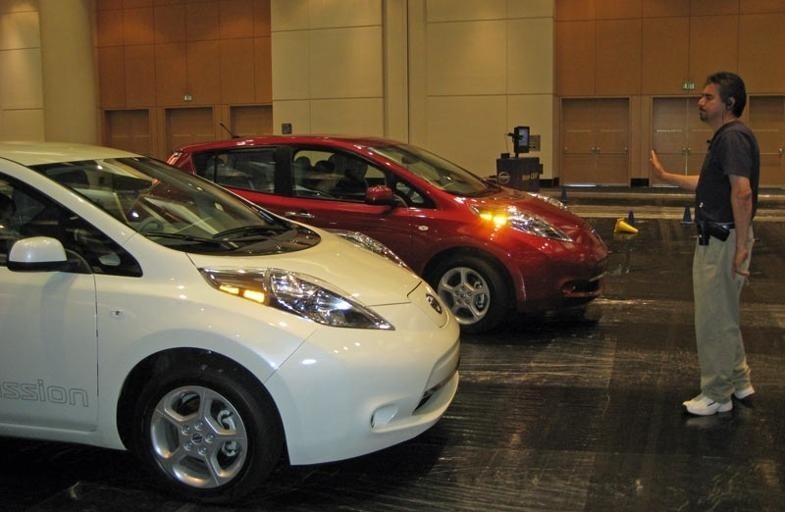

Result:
[679,202,693,225]
[613,211,641,233]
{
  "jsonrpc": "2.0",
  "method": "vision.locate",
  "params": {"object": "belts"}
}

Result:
[720,223,736,230]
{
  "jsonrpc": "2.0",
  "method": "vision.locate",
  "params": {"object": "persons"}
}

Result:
[0,191,106,275]
[648,71,762,417]
[332,156,370,197]
[29,169,104,258]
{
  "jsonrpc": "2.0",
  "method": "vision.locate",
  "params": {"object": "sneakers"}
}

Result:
[732,384,757,400]
[681,392,735,417]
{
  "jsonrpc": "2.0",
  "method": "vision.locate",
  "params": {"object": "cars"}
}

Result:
[127,136,608,337]
[1,138,462,506]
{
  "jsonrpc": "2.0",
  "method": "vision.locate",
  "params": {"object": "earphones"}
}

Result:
[727,99,732,106]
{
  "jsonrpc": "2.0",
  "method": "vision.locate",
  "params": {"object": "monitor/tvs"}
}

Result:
[513,126,530,158]
